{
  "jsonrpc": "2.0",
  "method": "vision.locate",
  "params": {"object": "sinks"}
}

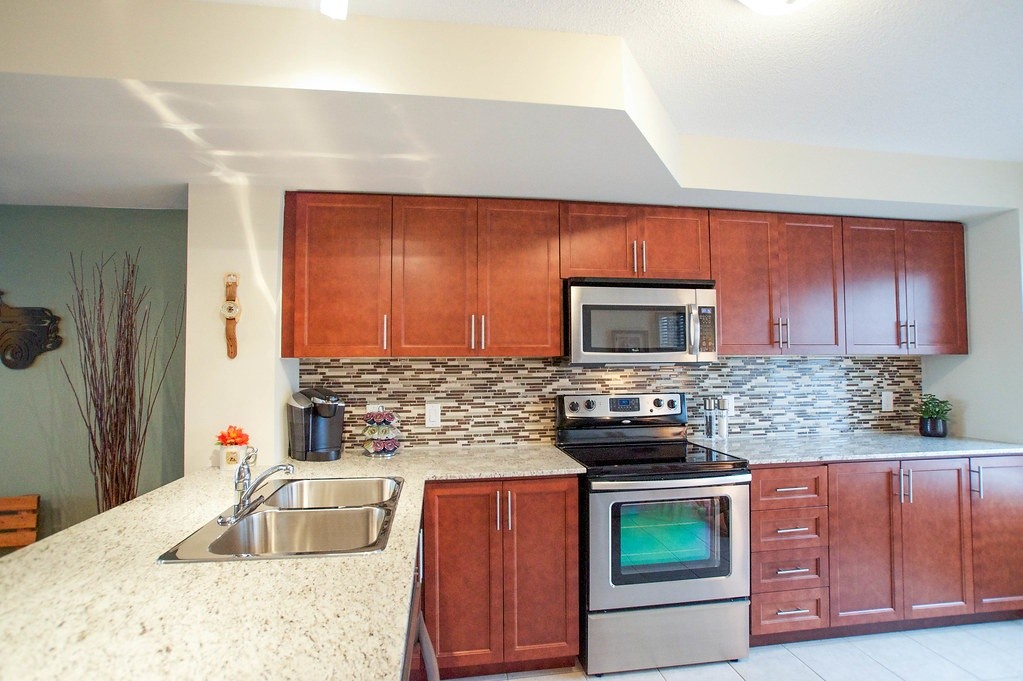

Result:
[229,476,405,509]
[156,507,397,565]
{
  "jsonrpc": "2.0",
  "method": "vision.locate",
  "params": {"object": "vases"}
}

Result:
[219,445,247,471]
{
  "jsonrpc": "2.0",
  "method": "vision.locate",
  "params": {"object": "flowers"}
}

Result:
[215,425,249,446]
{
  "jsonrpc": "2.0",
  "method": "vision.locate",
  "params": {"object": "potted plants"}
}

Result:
[912,392,953,437]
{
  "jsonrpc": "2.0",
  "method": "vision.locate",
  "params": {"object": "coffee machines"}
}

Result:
[287,388,346,462]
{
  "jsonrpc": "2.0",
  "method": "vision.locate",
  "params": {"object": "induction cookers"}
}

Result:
[554,392,750,477]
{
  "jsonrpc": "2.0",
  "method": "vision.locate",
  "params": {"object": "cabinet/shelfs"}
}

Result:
[829,458,970,639]
[560,199,711,283]
[403,475,581,681]
[281,190,392,358]
[710,209,845,356]
[843,213,969,355]
[749,465,829,649]
[391,194,563,359]
[970,455,1023,623]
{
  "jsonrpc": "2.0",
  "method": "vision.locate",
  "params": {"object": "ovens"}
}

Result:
[564,284,718,363]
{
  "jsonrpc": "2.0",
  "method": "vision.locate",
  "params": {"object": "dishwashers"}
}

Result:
[578,471,750,613]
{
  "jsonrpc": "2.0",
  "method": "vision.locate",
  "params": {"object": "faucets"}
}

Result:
[216,445,294,528]
